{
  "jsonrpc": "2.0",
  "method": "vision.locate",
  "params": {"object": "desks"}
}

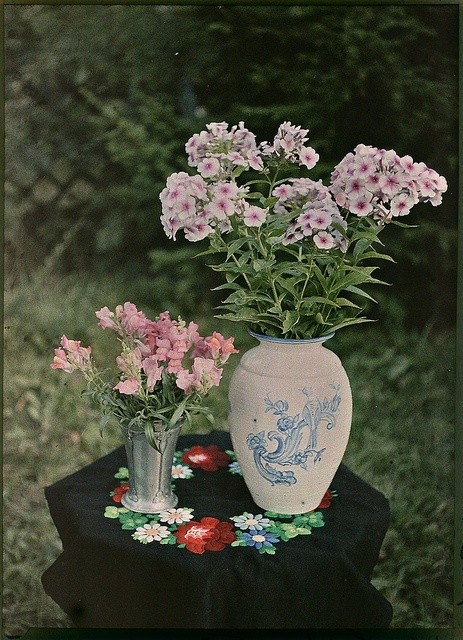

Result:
[47,431,389,629]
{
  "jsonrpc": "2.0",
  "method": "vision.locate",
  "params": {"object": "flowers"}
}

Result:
[157,117,448,336]
[52,301,240,454]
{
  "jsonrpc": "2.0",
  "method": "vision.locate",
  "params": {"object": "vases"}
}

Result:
[114,418,182,513]
[227,326,354,515]
